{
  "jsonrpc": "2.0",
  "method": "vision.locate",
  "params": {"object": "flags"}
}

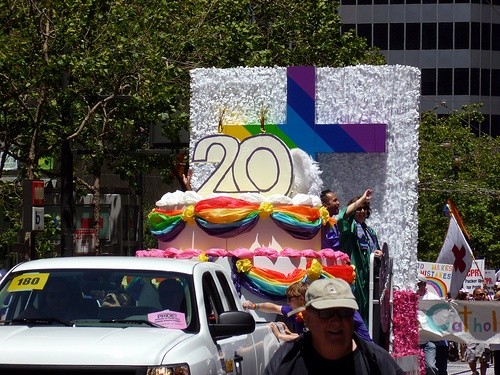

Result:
[435,216,474,300]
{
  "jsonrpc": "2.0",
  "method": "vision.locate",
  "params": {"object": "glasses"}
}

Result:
[310,310,356,320]
[356,206,367,211]
[287,295,303,302]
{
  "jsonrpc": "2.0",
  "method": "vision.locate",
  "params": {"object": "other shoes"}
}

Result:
[461,358,466,362]
[472,371,479,375]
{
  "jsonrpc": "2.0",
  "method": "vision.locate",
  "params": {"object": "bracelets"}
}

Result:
[255,303,260,311]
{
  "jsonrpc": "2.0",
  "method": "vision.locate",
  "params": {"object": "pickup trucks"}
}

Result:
[0,255,301,374]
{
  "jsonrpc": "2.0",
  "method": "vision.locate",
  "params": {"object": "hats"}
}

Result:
[305,277,359,312]
[417,273,428,283]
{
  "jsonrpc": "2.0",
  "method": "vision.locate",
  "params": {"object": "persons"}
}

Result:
[416,273,500,375]
[157,278,184,314]
[262,277,405,375]
[336,189,384,329]
[14,276,136,321]
[321,190,340,252]
[242,281,375,343]
